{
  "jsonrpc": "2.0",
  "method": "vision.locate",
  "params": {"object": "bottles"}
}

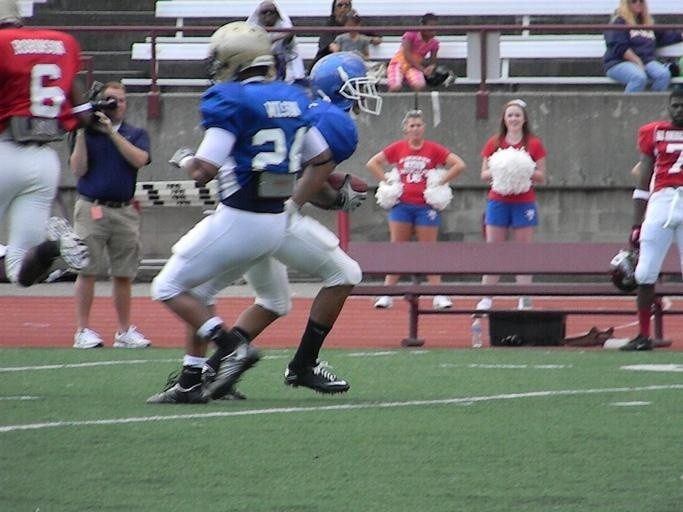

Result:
[472,318,483,348]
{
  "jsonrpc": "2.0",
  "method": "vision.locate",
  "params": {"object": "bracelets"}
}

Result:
[631,188,650,202]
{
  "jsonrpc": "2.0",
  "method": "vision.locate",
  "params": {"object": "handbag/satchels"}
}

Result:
[425,64,455,87]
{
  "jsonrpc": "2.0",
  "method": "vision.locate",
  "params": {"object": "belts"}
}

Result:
[79,195,130,207]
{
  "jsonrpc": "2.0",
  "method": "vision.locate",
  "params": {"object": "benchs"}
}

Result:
[120,0,683,93]
[343,241,683,347]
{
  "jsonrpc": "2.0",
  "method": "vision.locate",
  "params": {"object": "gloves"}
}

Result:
[169,145,195,167]
[630,225,641,247]
[335,175,367,213]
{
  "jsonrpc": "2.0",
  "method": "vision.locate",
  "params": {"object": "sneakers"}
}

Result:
[45,216,90,271]
[72,327,104,348]
[474,298,492,318]
[516,297,532,311]
[620,335,653,350]
[283,361,349,393]
[374,294,393,308]
[144,344,262,404]
[433,295,452,308]
[113,325,151,349]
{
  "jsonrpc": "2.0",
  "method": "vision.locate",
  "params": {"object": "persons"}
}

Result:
[145,19,312,403]
[203,50,369,401]
[364,109,466,311]
[68,80,153,350]
[470,96,547,319]
[244,0,306,86]
[599,0,683,92]
[307,0,352,76]
[385,12,440,94]
[0,0,91,288]
[333,8,382,113]
[618,90,683,350]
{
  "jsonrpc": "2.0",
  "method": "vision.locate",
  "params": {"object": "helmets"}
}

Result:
[309,49,385,118]
[203,20,276,85]
[608,251,638,291]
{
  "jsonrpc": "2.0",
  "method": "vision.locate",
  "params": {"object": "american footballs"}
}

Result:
[311,174,367,209]
